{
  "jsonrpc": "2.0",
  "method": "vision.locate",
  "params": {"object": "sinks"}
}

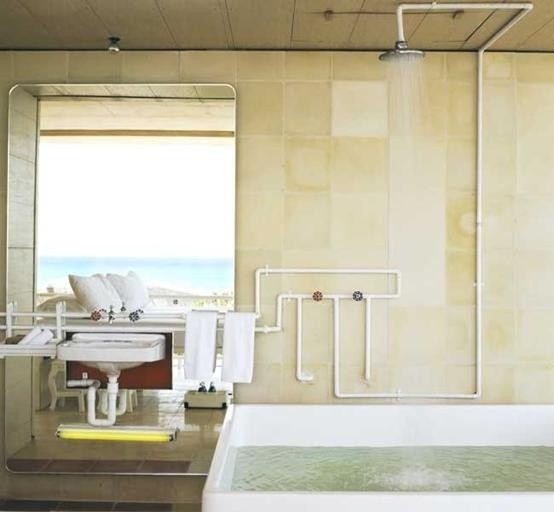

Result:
[56,332,167,374]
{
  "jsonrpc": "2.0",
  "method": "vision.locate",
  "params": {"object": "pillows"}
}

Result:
[106,271,150,311]
[68,272,123,315]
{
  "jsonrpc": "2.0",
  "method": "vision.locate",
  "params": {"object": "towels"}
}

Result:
[184,309,217,381]
[221,311,254,382]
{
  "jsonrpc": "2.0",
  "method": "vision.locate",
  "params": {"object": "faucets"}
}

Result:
[106,304,116,326]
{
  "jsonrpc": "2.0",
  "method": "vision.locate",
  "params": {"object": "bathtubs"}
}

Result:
[200,403,552,511]
